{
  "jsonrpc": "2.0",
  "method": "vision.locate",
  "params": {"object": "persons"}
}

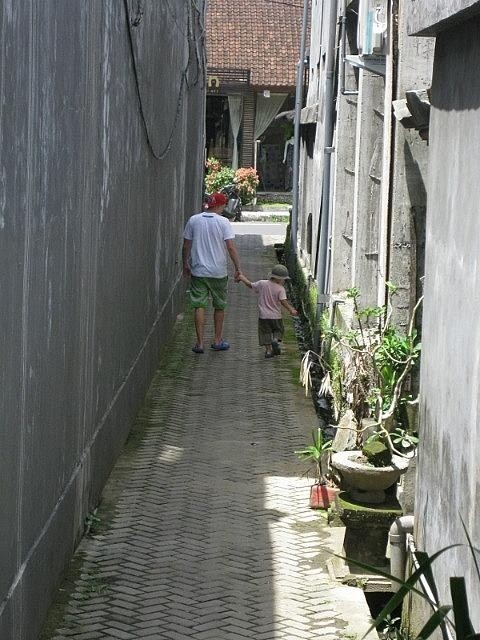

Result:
[182,192,242,353]
[236,265,298,358]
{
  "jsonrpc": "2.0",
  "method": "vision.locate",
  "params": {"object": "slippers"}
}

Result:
[210,340,230,350]
[192,346,204,353]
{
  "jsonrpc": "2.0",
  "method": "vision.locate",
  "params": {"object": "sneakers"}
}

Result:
[265,339,282,359]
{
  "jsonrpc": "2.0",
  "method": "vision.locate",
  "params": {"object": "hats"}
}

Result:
[267,265,292,280]
[204,192,227,210]
